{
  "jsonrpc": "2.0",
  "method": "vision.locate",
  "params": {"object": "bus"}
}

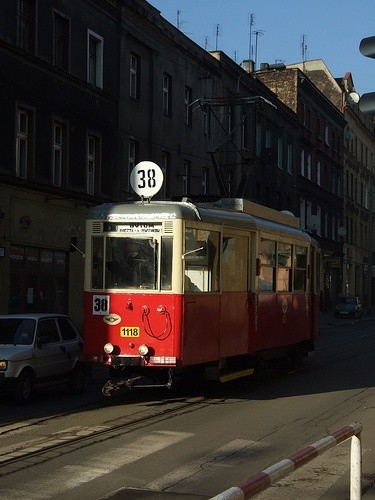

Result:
[67,195,321,389]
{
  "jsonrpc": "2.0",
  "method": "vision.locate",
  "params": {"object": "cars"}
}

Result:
[0,312,83,403]
[334,295,363,321]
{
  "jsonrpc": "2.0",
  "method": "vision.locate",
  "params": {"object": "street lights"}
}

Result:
[231,63,287,197]
